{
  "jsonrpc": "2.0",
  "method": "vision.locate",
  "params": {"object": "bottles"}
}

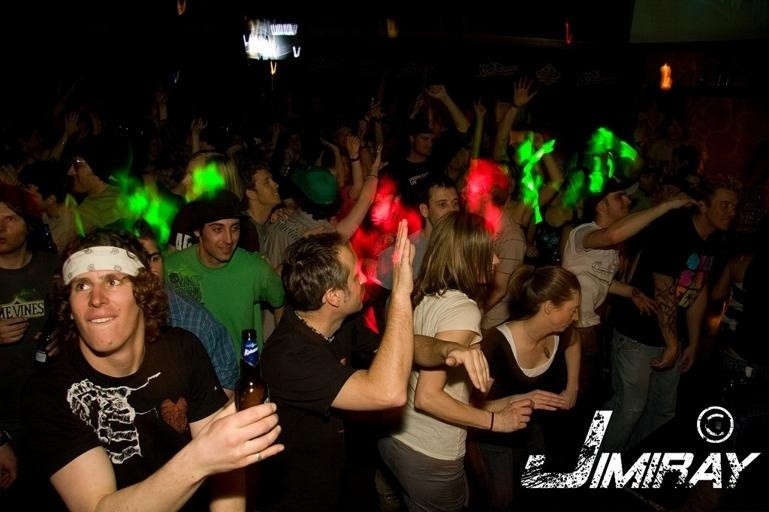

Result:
[35,309,54,371]
[234,329,270,412]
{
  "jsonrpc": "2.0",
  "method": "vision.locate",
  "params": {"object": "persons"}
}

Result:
[1,2,768,509]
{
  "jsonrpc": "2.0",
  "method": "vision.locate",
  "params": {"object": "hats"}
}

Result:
[582,175,641,214]
[185,189,252,234]
[298,167,339,206]
